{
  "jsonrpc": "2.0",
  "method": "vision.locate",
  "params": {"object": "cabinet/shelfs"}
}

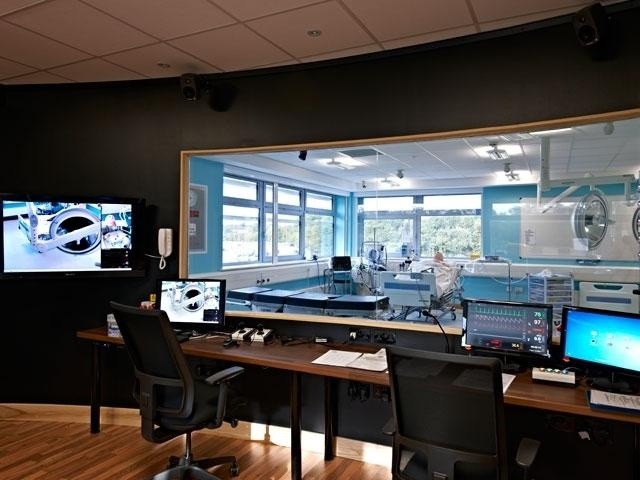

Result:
[528,273,575,322]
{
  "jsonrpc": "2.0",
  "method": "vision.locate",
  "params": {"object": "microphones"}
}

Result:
[423,309,450,353]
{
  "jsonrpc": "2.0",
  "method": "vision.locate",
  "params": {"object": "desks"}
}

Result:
[77,326,640,480]
[228,286,390,320]
[324,268,374,294]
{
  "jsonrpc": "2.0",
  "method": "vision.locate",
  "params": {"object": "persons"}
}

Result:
[435,252,448,268]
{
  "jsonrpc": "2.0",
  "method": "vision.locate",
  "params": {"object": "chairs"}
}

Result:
[331,256,352,294]
[108,301,244,480]
[382,345,541,480]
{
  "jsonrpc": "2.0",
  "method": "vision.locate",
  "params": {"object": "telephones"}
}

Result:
[157,227,174,258]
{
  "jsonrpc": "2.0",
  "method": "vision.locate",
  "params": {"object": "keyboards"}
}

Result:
[175,335,188,342]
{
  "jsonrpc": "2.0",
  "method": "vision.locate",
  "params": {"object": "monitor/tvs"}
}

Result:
[557,305,640,393]
[0,196,147,279]
[156,279,226,339]
[462,298,552,373]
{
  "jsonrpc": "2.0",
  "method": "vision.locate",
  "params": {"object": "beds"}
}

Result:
[379,269,462,324]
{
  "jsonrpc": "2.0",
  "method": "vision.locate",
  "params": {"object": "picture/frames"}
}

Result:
[188,183,207,254]
